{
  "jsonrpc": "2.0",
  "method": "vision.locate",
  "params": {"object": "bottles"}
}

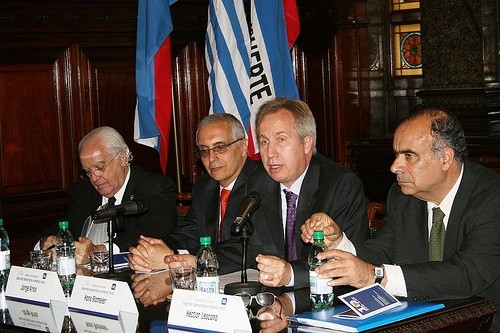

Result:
[0,219,10,321]
[194,236,220,294]
[307,230,334,310]
[55,221,77,295]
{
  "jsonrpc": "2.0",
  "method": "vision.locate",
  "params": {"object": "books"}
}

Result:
[297,283,445,333]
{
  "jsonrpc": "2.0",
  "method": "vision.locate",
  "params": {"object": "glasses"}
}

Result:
[195,138,244,159]
[81,150,121,180]
[234,291,283,306]
[244,306,283,321]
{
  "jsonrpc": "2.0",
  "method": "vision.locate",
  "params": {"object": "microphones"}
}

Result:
[231,190,262,236]
[91,199,149,220]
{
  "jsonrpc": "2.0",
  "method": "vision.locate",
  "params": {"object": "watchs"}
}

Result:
[373,264,384,284]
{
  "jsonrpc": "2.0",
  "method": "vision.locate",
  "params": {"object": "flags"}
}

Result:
[206,0,302,161]
[134,1,173,172]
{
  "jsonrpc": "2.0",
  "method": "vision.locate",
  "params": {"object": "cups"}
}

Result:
[30,250,52,271]
[167,266,194,291]
[90,251,110,274]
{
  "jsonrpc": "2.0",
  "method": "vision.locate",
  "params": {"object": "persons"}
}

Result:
[257,286,352,333]
[127,113,279,272]
[32,126,176,264]
[164,97,369,291]
[130,270,175,333]
[300,108,500,333]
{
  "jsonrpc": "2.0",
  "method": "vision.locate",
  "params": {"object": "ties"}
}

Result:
[219,188,232,242]
[107,196,120,242]
[283,189,298,262]
[429,207,446,262]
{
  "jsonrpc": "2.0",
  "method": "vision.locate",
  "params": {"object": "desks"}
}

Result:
[10,252,500,333]
